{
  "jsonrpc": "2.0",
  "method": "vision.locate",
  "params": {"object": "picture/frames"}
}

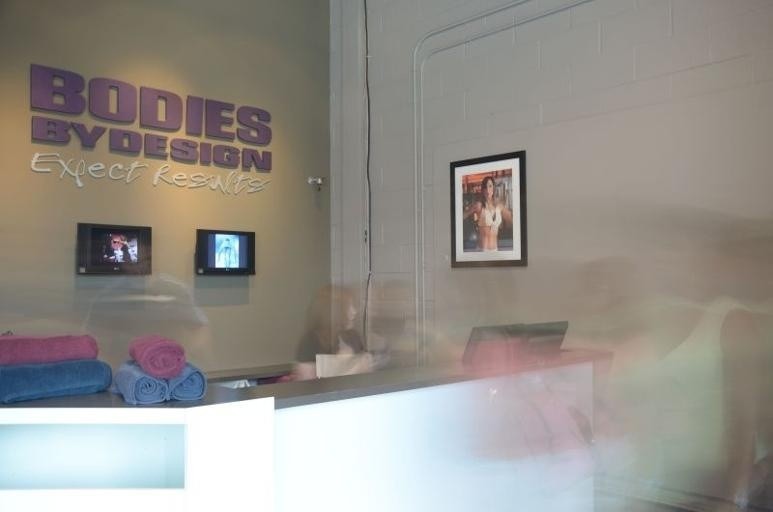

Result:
[449,150,527,268]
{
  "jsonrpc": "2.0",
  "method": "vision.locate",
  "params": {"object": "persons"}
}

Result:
[295,286,391,381]
[217,237,238,271]
[103,231,134,269]
[460,178,514,257]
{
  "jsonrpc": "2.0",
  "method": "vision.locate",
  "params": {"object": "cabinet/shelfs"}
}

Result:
[0,349,612,512]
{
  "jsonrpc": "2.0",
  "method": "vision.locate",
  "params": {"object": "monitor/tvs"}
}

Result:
[460,319,572,370]
[194,229,256,276]
[75,223,151,275]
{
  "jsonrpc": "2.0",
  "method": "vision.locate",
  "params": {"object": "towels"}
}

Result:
[0,334,100,365]
[116,360,168,405]
[0,360,112,405]
[128,333,186,376]
[169,363,206,402]
